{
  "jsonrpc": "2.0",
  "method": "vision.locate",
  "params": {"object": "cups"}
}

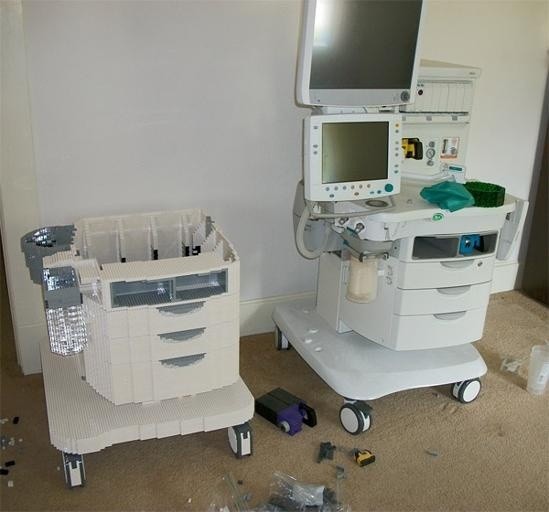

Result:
[526,343,549,398]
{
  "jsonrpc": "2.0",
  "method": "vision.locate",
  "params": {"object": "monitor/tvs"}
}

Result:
[295,0,425,108]
[304,113,402,202]
